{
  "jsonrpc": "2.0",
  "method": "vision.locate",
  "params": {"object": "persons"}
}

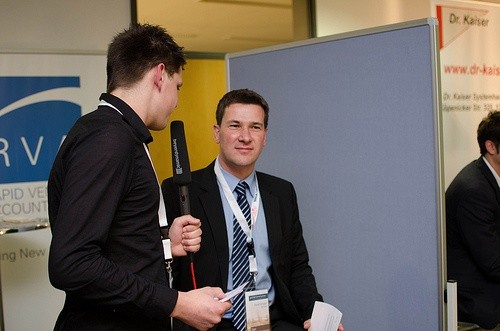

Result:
[445,109,500,331]
[47,23,232,331]
[161,89,344,331]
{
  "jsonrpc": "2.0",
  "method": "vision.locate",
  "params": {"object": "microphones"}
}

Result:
[170,120,194,263]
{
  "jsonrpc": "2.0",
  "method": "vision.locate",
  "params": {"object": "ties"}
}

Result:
[232,181,256,331]
